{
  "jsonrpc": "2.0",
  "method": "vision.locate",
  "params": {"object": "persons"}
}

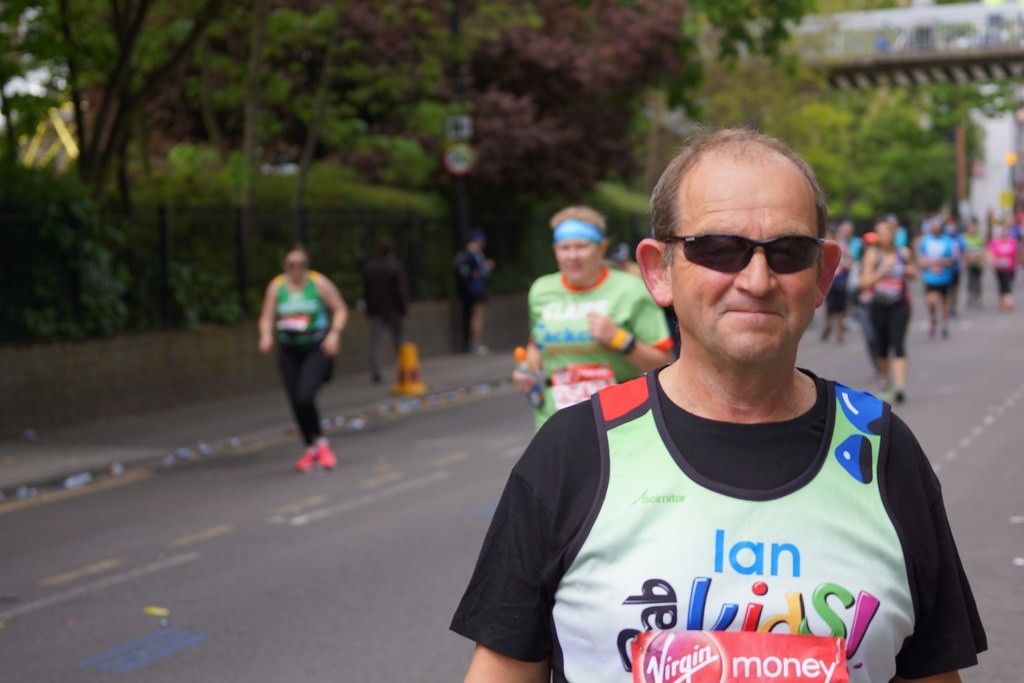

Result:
[960,217,986,311]
[822,197,965,402]
[453,230,496,358]
[994,217,1018,311]
[258,245,350,475]
[447,128,990,683]
[510,204,675,436]
[362,238,412,384]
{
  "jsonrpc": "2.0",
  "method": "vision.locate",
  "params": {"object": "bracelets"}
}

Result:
[611,328,636,355]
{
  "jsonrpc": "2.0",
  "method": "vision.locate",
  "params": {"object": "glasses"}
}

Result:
[658,234,825,272]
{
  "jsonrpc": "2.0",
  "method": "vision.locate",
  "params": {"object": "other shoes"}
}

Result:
[821,327,831,340]
[928,321,935,336]
[315,443,337,468]
[295,447,319,471]
[942,328,948,338]
[836,328,845,342]
[999,297,1013,310]
[893,390,905,403]
